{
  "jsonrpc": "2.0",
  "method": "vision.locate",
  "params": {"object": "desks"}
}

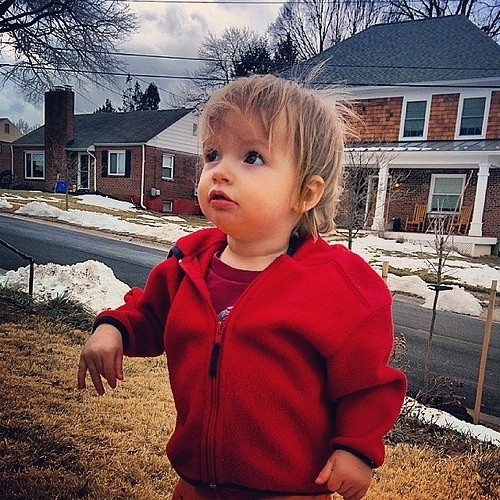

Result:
[425,215,447,235]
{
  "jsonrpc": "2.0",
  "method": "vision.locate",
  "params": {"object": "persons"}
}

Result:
[77,75,408,500]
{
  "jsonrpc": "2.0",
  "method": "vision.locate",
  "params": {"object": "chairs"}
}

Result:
[446,203,473,233]
[405,203,427,232]
